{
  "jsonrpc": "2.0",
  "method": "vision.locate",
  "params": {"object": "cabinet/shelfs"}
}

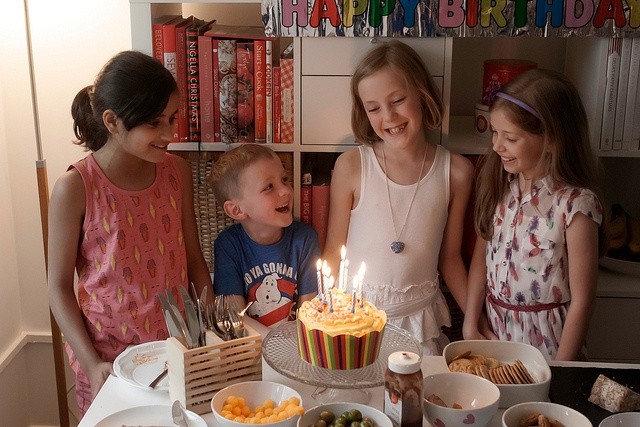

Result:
[129,0,639,364]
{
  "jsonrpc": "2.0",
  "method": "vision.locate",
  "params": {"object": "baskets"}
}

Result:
[189,160,293,272]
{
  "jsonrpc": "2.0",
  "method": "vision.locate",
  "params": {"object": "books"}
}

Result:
[312,185,329,256]
[153,15,293,143]
[301,187,312,227]
[168,151,239,272]
[601,36,639,151]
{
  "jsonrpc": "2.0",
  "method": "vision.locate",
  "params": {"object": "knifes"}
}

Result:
[159,281,208,349]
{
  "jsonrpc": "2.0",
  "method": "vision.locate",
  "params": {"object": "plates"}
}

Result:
[113,340,169,392]
[93,405,208,426]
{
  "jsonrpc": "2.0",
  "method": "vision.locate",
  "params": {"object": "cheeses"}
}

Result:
[587,374,640,413]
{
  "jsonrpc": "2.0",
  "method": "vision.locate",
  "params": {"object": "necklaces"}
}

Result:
[382,138,429,253]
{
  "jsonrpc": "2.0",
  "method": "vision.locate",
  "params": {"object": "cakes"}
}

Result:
[295,285,388,370]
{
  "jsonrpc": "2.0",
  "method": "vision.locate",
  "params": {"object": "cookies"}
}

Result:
[447,349,536,384]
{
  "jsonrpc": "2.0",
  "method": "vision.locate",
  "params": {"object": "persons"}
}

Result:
[48,51,215,422]
[210,144,320,329]
[320,39,500,356]
[462,68,603,361]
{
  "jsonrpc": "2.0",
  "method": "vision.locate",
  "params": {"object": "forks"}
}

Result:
[206,294,257,341]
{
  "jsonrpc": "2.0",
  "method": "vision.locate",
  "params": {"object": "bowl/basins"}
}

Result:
[211,380,303,426]
[501,401,593,426]
[443,339,552,409]
[423,372,501,426]
[297,402,393,426]
